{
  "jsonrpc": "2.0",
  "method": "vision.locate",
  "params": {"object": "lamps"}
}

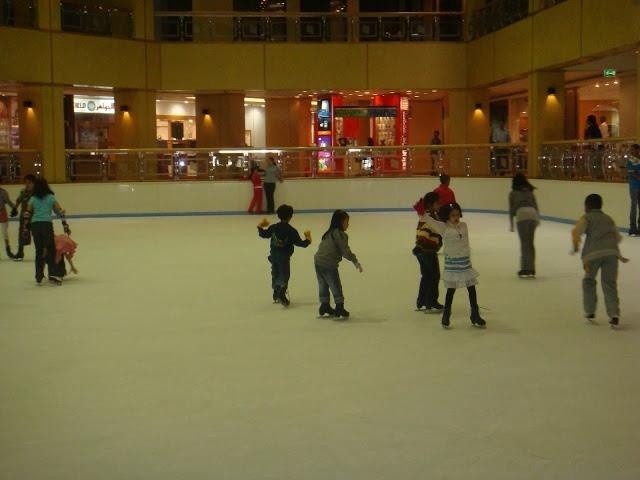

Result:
[473,103,482,111]
[24,100,33,109]
[201,108,210,116]
[546,86,556,96]
[120,104,129,113]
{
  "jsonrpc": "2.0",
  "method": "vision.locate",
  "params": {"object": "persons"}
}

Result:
[0,188,18,259]
[366,138,375,146]
[491,119,509,169]
[571,193,629,325]
[313,209,362,318]
[626,143,640,237]
[583,115,604,149]
[12,175,36,260]
[598,116,609,148]
[432,174,456,205]
[414,198,486,329]
[257,205,312,306]
[22,175,72,288]
[412,192,445,312]
[508,173,541,278]
[261,157,284,215]
[430,130,442,176]
[246,167,263,214]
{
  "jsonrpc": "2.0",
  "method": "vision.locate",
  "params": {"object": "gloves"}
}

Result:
[568,246,578,254]
[303,231,311,241]
[258,218,270,228]
[10,208,18,217]
[413,198,425,216]
[22,229,29,238]
[412,246,424,256]
[63,224,71,235]
[354,262,362,272]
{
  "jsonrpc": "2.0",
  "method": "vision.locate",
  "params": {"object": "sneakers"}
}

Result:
[275,286,289,304]
[335,304,349,317]
[518,270,527,275]
[426,300,443,308]
[417,299,428,308]
[6,250,15,258]
[586,314,595,318]
[49,272,64,277]
[320,302,334,314]
[35,273,44,281]
[15,248,23,258]
[609,318,618,325]
[273,289,279,300]
[470,315,485,325]
[528,270,535,275]
[442,316,449,326]
